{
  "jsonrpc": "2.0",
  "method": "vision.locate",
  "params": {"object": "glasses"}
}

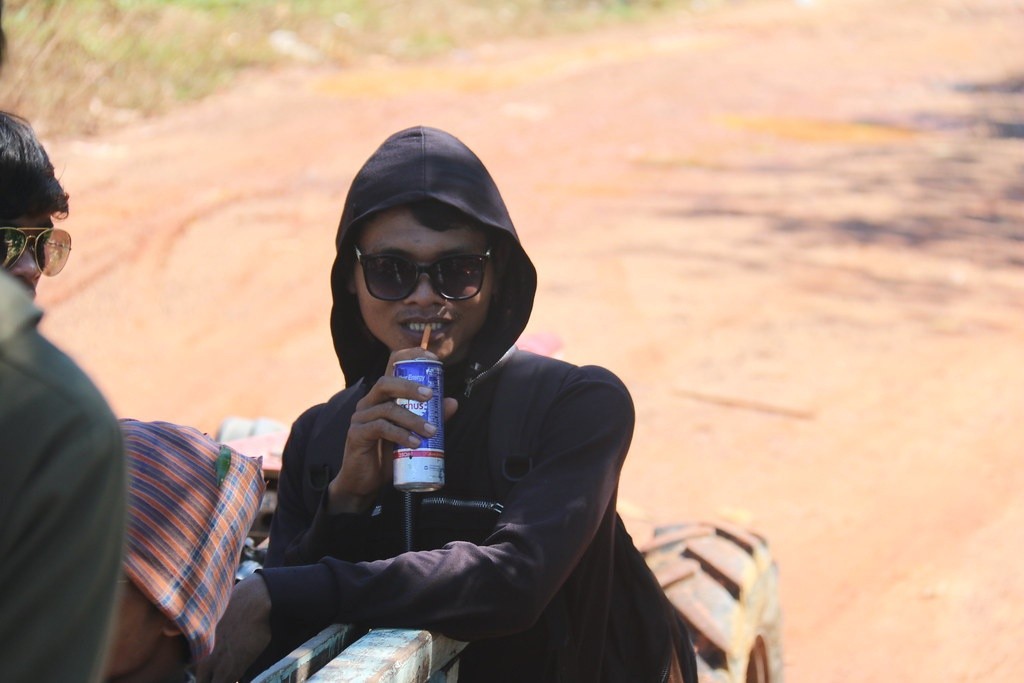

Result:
[355,244,493,301]
[0,227,71,277]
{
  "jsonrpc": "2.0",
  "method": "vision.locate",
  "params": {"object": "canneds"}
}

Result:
[394,360,445,490]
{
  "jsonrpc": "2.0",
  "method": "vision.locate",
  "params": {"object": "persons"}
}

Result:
[188,125,699,683]
[103,417,267,683]
[0,0,130,683]
[0,110,69,301]
